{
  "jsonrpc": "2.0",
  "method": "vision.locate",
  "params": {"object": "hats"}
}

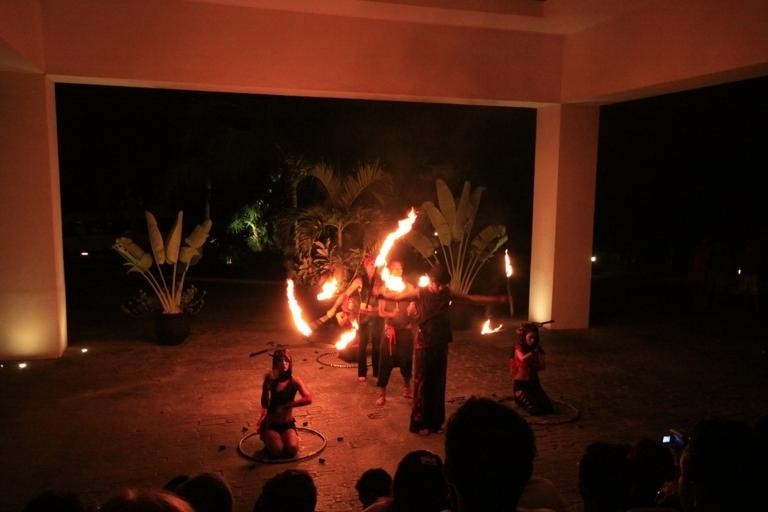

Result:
[427,264,450,284]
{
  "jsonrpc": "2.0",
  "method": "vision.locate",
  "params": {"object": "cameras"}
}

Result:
[661,434,672,444]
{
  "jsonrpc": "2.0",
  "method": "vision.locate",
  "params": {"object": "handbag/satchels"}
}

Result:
[509,359,530,380]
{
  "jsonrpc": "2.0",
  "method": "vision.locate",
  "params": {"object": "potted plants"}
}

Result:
[282,157,509,364]
[105,208,215,345]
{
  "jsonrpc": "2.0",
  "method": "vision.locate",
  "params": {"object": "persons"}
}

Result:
[336,299,361,363]
[511,323,556,418]
[256,348,313,460]
[372,262,508,439]
[326,252,379,384]
[375,260,415,406]
